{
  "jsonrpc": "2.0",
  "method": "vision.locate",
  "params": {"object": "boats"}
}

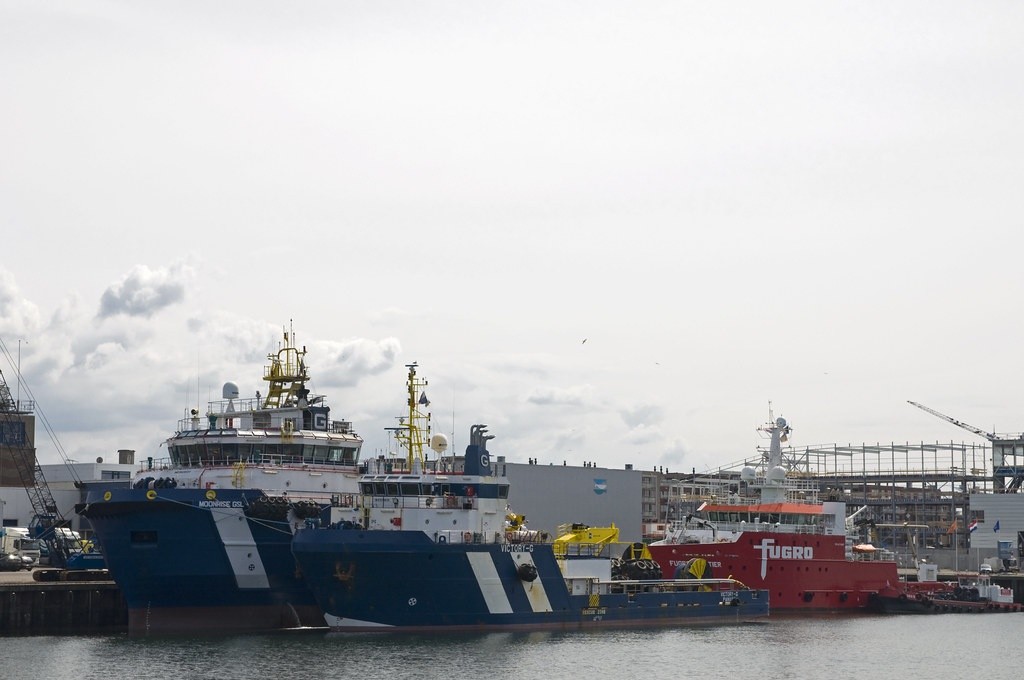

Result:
[75,390,364,634]
[645,504,1023,613]
[289,471,771,635]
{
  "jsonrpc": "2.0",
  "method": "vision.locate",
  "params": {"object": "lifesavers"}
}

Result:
[446,496,454,505]
[899,593,1024,614]
[643,560,654,570]
[871,592,879,600]
[840,592,848,599]
[651,560,661,569]
[804,592,813,601]
[506,532,512,539]
[635,561,646,570]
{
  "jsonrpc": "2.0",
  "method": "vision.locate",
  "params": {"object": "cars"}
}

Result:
[981,563,994,574]
[0,554,36,571]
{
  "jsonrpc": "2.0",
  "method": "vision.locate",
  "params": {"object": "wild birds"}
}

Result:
[582,339,588,344]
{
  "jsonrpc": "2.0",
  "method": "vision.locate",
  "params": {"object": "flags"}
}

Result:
[992,520,1000,533]
[947,520,956,534]
[968,520,977,532]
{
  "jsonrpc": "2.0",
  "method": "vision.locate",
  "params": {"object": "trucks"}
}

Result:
[2,524,84,558]
